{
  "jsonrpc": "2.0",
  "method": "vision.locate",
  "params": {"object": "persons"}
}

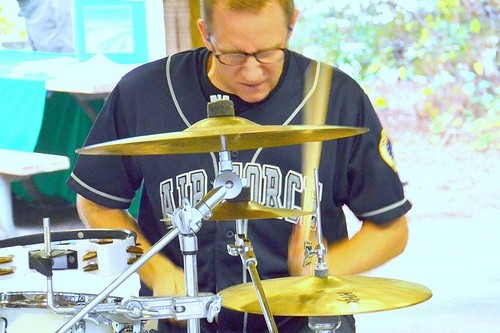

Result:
[67,0,412,332]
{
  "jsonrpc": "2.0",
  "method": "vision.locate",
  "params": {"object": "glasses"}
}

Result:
[205,27,292,66]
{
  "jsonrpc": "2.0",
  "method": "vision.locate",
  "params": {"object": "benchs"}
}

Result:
[0,149,69,234]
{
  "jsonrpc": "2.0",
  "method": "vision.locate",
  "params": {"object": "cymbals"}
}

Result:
[75,114,370,156]
[215,269,431,318]
[199,201,315,221]
[0,228,142,287]
